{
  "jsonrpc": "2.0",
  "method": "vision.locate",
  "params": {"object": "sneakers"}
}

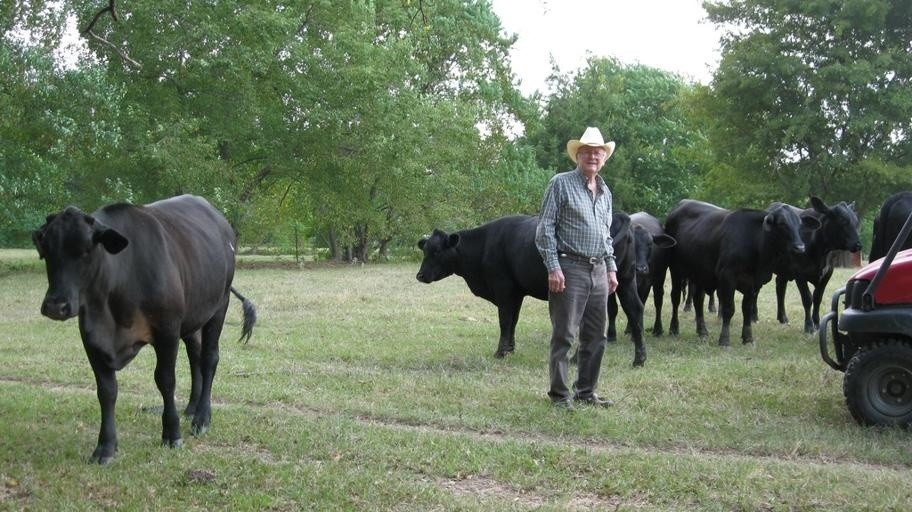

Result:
[574,393,614,408]
[550,396,577,415]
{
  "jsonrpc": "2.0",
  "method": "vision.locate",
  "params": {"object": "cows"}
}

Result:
[30,193,257,466]
[750,196,863,336]
[664,198,822,350]
[623,211,678,342]
[416,210,647,367]
[868,191,912,263]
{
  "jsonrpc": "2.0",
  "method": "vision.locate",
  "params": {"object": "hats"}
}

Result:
[566,126,616,164]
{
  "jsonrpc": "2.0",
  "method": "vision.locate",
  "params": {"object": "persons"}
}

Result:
[535,126,620,409]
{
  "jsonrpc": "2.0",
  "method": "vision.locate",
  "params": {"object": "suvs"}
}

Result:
[820,212,911,433]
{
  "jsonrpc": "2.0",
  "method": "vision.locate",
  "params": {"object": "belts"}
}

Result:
[557,252,605,265]
